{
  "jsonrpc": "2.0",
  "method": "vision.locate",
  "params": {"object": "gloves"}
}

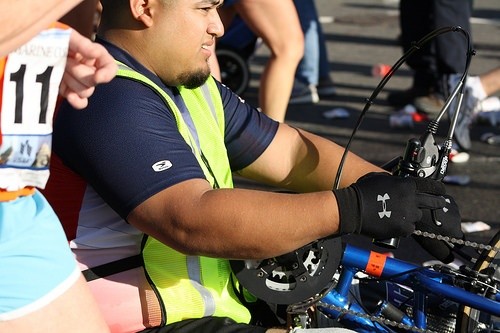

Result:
[332,172,445,241]
[411,195,465,264]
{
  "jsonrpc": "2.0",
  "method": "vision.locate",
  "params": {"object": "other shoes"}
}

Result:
[317,74,336,95]
[414,94,447,115]
[387,86,439,106]
[289,85,319,104]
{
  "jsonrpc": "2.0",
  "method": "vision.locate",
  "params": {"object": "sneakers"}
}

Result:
[442,73,479,151]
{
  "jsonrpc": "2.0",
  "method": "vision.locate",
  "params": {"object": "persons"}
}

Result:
[38,0,454,332]
[205,0,304,124]
[1,1,119,333]
[386,0,473,133]
[214,0,355,120]
[441,65,500,152]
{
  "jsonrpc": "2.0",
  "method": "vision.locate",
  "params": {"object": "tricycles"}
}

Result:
[228,26,500,333]
